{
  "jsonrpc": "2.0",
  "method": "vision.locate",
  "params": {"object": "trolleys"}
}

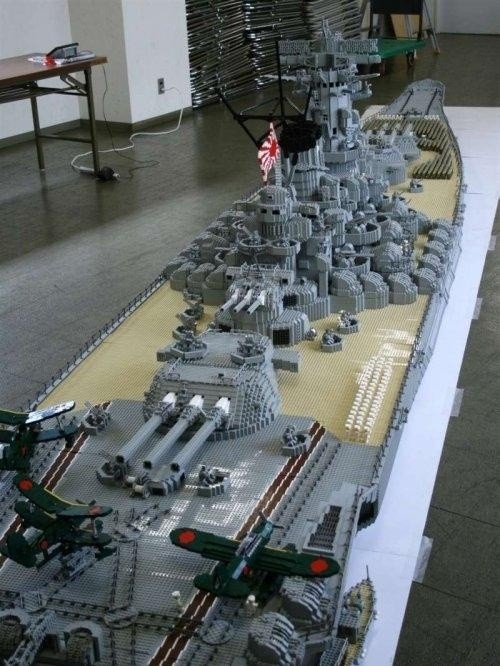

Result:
[369,0,424,76]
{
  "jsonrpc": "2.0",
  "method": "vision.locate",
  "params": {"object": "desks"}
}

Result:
[0,52,110,177]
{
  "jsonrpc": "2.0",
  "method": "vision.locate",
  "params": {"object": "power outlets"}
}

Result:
[157,77,165,94]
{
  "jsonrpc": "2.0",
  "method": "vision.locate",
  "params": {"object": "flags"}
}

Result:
[256,121,283,183]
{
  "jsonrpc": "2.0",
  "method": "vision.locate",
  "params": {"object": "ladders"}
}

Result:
[405,0,440,59]
